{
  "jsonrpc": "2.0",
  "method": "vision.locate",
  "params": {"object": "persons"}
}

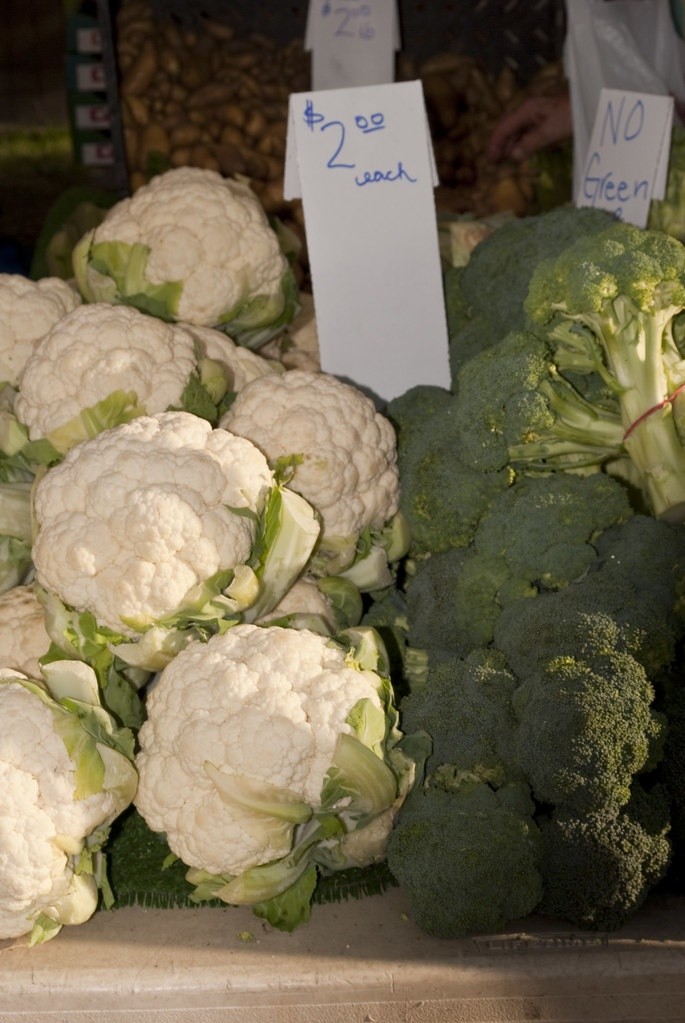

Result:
[488,0,685,207]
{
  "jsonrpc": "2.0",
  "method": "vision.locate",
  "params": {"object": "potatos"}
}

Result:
[114,0,567,223]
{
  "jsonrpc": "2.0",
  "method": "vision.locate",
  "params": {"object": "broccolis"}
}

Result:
[0,117,685,941]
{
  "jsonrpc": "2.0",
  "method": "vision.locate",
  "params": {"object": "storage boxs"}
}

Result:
[0,1,118,194]
